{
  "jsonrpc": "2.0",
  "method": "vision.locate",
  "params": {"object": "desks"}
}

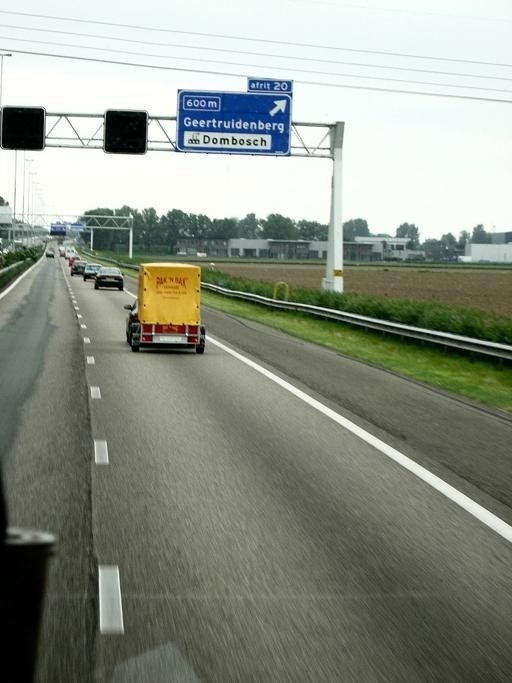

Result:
[23,156,41,249]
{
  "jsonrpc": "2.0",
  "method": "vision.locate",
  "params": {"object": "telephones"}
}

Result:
[175,77,294,157]
[50,225,66,236]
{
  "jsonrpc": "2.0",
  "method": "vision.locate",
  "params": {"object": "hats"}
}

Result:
[59,246,88,277]
[46,250,55,258]
[95,266,123,291]
[83,263,102,282]
